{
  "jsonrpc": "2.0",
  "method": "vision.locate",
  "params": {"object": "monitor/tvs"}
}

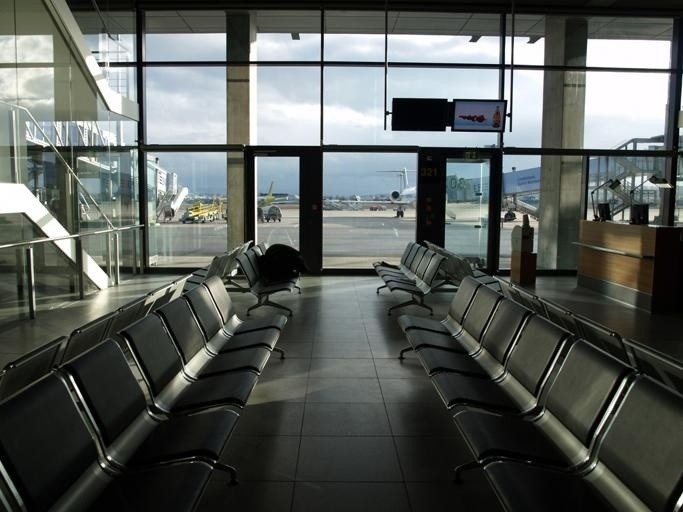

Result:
[451,99,507,132]
[391,98,448,131]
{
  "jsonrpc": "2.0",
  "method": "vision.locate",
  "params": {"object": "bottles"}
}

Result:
[492,106,501,129]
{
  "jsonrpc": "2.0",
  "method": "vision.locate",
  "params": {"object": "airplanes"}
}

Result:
[187,180,290,223]
[323,168,455,221]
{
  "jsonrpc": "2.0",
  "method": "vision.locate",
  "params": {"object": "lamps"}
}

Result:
[591,177,623,221]
[630,176,673,220]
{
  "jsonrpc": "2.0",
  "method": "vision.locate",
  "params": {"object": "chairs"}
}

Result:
[372,239,683,511]
[0,240,302,512]
